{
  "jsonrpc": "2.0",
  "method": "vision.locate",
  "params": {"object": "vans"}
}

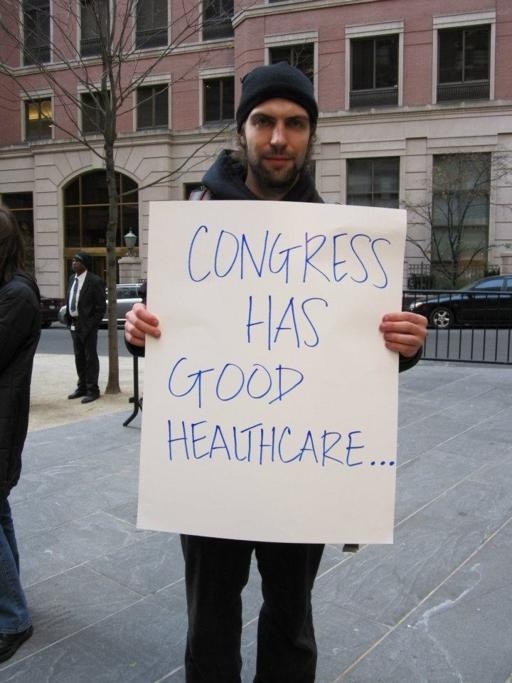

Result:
[59,283,143,329]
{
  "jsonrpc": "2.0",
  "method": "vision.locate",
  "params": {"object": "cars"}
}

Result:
[40,296,65,328]
[411,274,511,327]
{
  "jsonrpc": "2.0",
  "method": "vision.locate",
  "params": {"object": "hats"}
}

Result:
[235,60,319,132]
[74,252,92,270]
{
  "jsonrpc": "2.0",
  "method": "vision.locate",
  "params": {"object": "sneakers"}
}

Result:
[68,391,100,403]
[0,624,33,663]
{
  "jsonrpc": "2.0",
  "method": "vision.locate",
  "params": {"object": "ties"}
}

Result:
[70,279,78,310]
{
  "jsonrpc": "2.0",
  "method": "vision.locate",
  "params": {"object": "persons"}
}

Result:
[64,252,107,403]
[120,59,432,682]
[1,198,43,659]
